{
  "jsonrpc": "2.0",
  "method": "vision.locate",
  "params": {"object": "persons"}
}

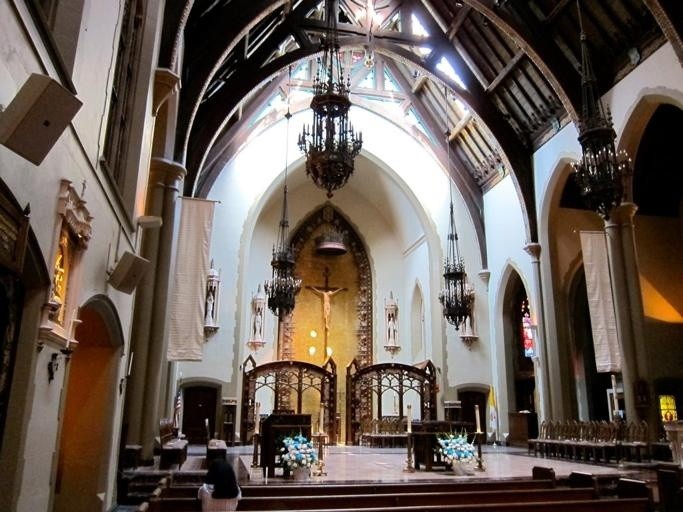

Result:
[196,457,243,511]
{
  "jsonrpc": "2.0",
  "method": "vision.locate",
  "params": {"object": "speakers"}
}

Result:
[109,250,151,295]
[0,72,83,167]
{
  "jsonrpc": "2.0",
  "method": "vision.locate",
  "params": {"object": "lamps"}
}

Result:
[262,63,302,321]
[570,0,634,221]
[437,84,473,330]
[295,1,362,198]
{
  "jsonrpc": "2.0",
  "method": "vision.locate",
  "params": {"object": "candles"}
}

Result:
[475,405,482,433]
[407,403,412,433]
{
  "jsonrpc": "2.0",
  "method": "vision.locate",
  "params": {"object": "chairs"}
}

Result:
[527,418,651,467]
[363,416,409,449]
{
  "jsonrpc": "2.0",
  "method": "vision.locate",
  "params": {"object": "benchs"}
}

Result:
[159,419,188,470]
[122,467,683,511]
[208,439,228,464]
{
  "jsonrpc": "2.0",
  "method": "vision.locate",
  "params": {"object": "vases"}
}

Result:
[453,461,472,474]
[293,467,310,482]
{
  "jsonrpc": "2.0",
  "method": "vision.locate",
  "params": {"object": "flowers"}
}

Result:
[279,428,318,468]
[436,424,482,464]
